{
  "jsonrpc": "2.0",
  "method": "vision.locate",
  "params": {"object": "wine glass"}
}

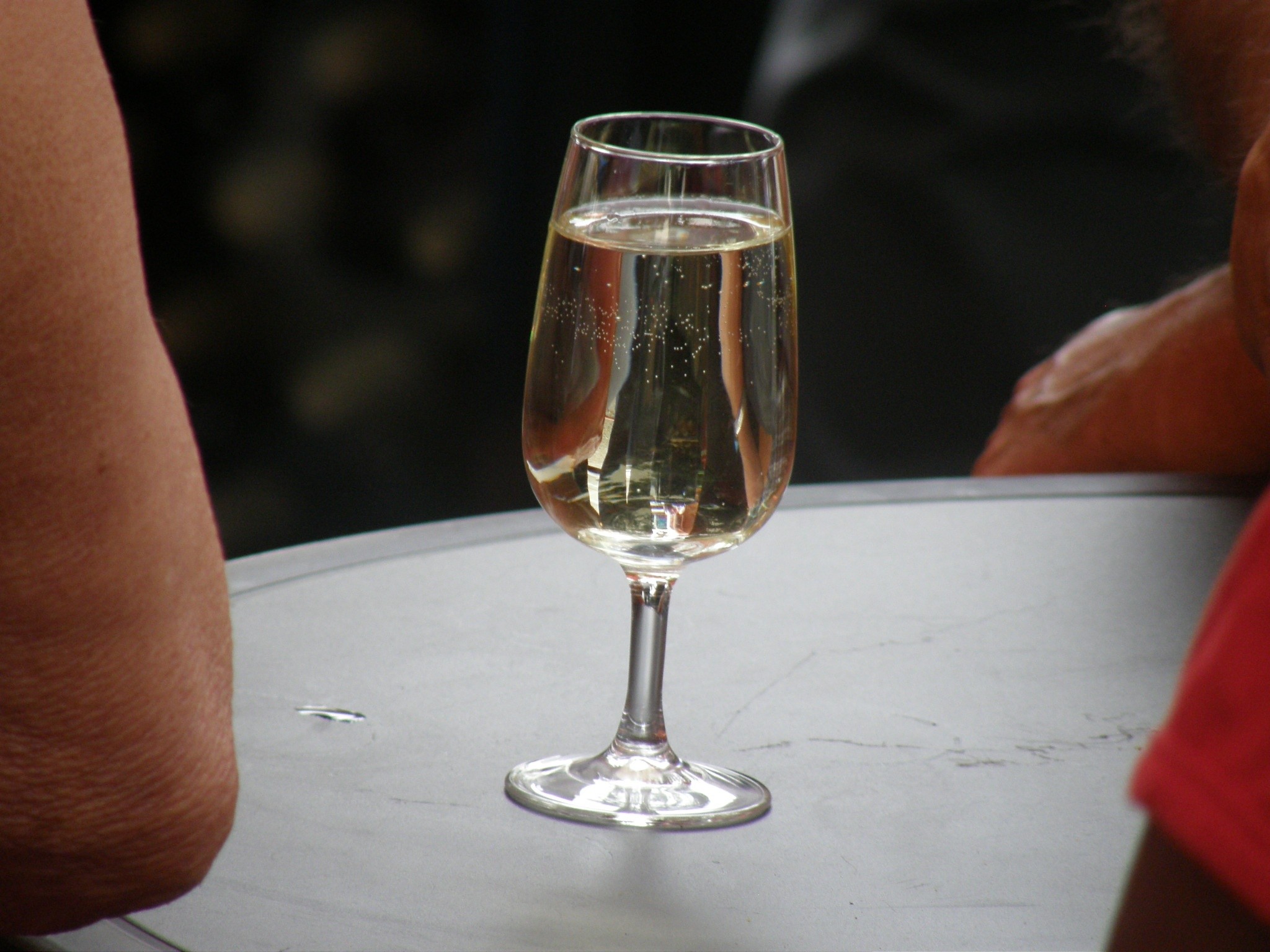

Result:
[504,108,802,835]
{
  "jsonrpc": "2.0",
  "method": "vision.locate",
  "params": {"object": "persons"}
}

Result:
[0,0,236,934]
[0,0,1270,952]
